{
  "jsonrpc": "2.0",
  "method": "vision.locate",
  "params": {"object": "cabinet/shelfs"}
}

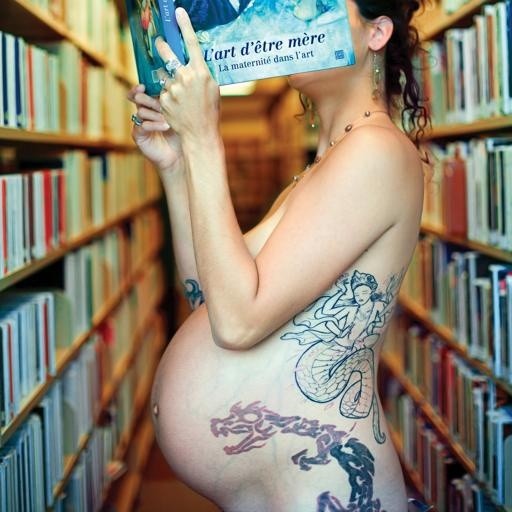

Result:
[0,0,173,512]
[304,0,512,512]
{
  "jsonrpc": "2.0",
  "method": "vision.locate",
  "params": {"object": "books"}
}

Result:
[379,0,511,511]
[126,1,357,98]
[1,1,172,511]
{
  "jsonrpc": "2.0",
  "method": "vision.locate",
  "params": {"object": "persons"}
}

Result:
[127,0,432,512]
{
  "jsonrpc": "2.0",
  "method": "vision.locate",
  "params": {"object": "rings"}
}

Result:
[165,57,183,78]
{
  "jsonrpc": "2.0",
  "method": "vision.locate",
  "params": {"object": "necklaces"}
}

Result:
[291,108,388,186]
